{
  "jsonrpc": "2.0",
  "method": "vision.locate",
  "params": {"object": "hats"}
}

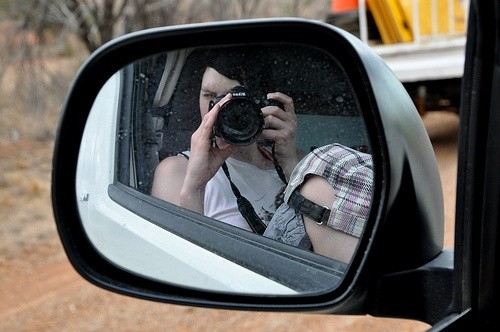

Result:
[283,143,374,239]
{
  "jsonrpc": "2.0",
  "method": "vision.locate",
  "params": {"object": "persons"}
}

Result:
[151,46,373,265]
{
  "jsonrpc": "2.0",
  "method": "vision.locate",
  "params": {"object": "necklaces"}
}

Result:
[256,157,267,167]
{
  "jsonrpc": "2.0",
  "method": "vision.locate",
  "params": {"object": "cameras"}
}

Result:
[213,85,277,147]
